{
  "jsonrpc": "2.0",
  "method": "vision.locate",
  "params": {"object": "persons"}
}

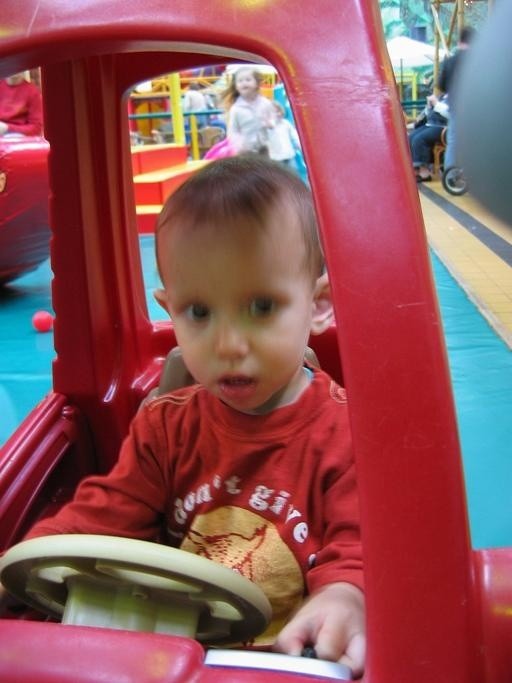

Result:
[226,68,275,157]
[437,26,478,172]
[265,99,301,169]
[179,82,208,127]
[21,153,369,681]
[0,69,43,136]
[405,88,450,183]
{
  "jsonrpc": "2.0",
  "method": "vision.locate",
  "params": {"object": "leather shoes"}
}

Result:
[416,173,434,183]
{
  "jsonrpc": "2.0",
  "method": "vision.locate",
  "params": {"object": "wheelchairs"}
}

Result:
[432,127,468,196]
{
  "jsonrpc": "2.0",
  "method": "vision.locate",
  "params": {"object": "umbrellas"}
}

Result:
[384,32,454,101]
